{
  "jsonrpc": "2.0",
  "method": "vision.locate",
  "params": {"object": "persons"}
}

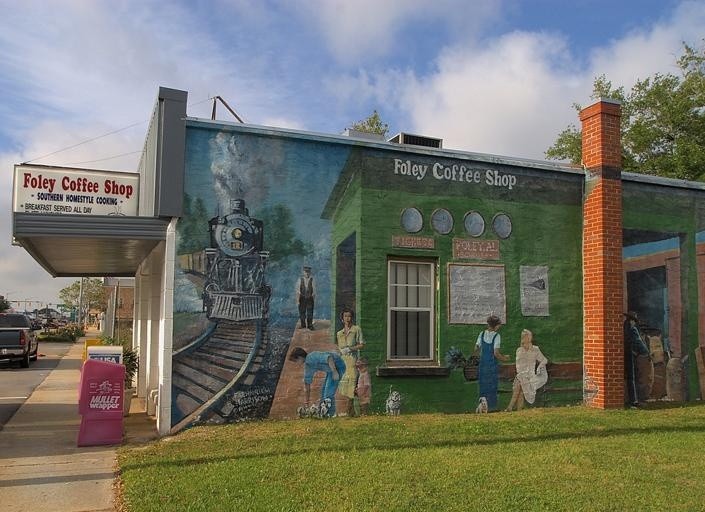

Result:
[474,315,510,412]
[505,329,548,412]
[632,332,655,400]
[354,360,372,416]
[294,266,316,331]
[336,308,366,416]
[288,348,346,419]
[624,310,650,406]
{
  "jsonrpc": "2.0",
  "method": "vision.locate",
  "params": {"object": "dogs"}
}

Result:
[296,404,318,418]
[319,398,332,418]
[475,396,489,415]
[385,384,402,416]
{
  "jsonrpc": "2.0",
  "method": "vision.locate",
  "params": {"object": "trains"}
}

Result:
[174,199,273,322]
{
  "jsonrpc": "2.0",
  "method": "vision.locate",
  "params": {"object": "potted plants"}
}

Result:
[341,111,391,140]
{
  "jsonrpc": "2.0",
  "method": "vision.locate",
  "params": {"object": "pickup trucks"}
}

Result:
[1,312,37,368]
